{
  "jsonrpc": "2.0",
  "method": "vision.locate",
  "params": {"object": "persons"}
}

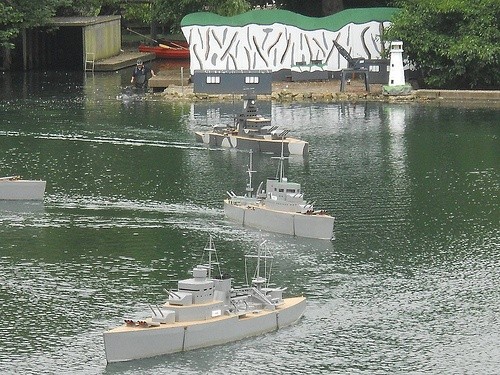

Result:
[130,59,156,89]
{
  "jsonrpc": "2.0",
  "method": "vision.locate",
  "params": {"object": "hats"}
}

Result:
[137,59,143,65]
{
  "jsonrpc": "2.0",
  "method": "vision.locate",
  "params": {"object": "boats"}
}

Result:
[195,86,309,155]
[223,136,335,240]
[0,177,47,201]
[103,235,308,364]
[139,39,190,59]
